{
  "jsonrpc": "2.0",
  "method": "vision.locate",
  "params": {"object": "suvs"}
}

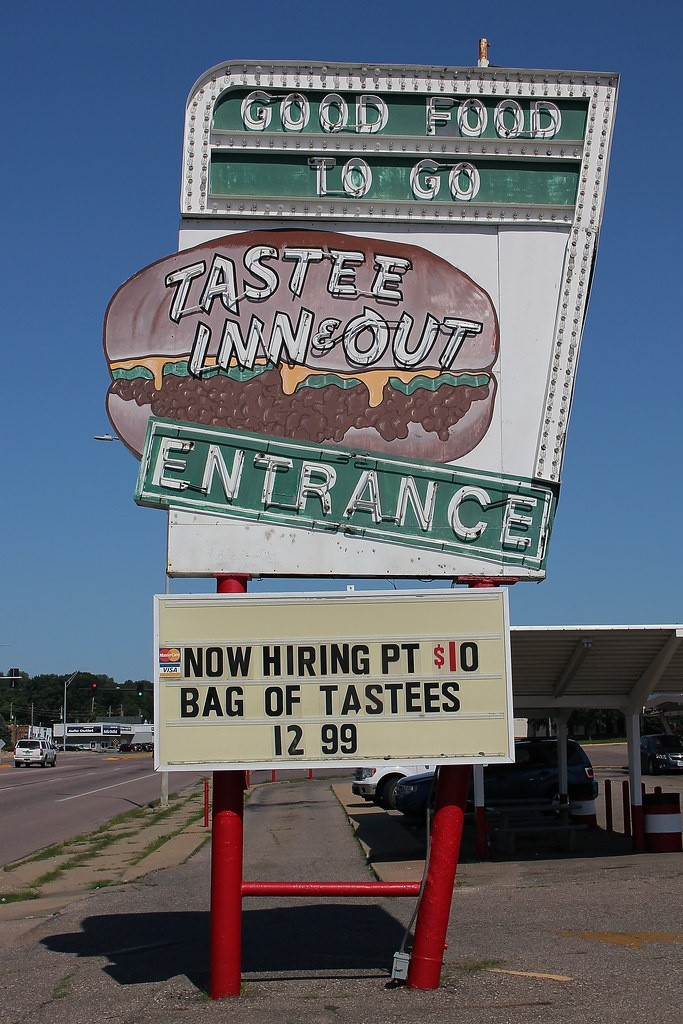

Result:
[393,739,600,825]
[14,738,57,767]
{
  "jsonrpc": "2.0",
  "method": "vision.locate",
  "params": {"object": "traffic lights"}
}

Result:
[90,682,97,696]
[136,683,144,697]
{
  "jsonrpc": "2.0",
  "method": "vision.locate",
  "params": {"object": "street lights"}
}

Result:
[64,664,89,750]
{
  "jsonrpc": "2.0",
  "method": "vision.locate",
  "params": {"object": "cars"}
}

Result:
[101,746,119,754]
[119,743,154,753]
[640,734,683,774]
